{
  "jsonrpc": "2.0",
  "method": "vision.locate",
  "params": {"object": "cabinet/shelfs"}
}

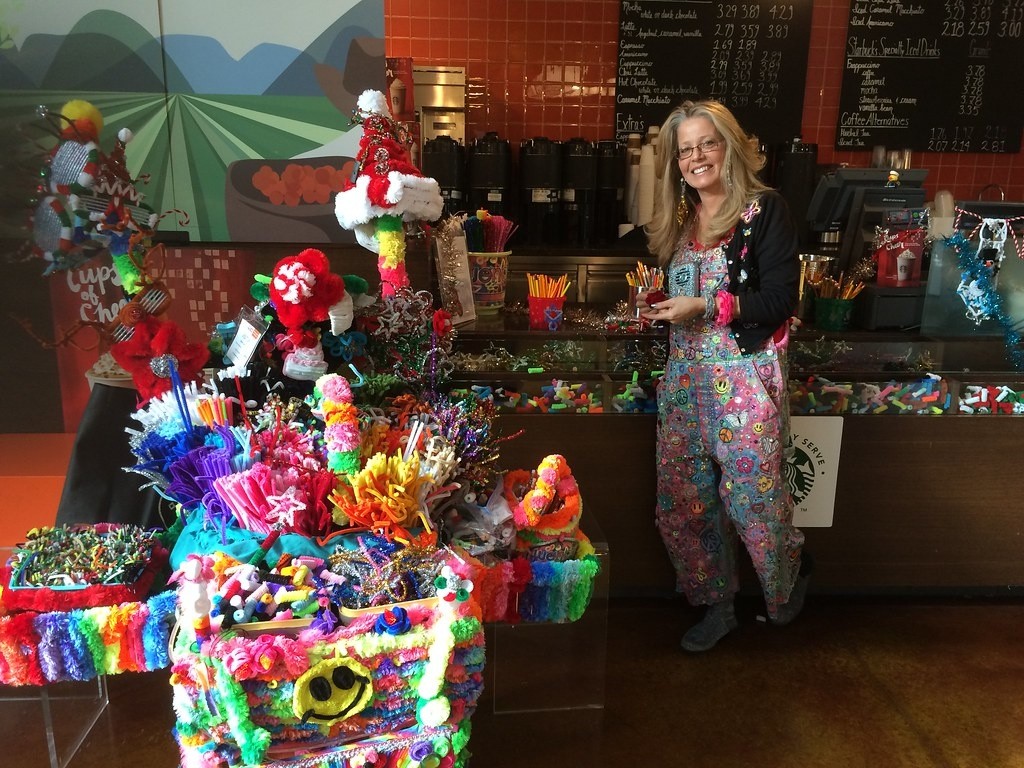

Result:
[429,250,1024,605]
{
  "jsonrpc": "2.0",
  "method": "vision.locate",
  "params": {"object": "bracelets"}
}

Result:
[702,293,715,321]
[716,290,734,327]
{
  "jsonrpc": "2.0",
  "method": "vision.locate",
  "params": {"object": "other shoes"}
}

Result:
[772,550,812,625]
[682,606,739,652]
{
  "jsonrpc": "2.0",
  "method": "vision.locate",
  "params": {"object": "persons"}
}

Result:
[636,100,805,652]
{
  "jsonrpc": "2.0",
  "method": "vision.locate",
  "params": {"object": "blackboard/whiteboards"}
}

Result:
[616,0,813,143]
[833,0,1024,156]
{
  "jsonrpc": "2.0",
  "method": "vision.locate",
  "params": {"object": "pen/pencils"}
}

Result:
[525,272,571,297]
[804,268,865,301]
[625,260,665,288]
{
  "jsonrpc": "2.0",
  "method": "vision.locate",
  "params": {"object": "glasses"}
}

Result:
[671,138,729,160]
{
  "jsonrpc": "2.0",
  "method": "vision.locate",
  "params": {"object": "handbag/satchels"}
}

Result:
[920,206,1024,340]
